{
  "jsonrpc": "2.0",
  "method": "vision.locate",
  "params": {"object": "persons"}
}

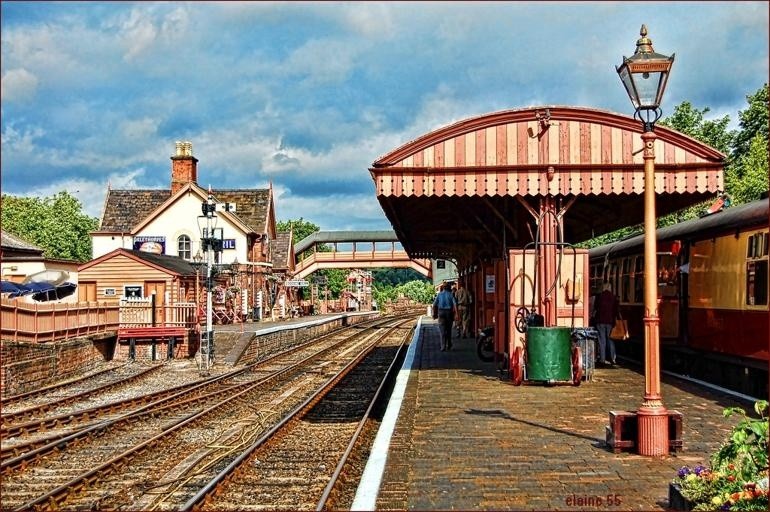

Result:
[432,283,459,352]
[455,281,472,339]
[452,287,456,297]
[590,287,597,323]
[592,282,621,364]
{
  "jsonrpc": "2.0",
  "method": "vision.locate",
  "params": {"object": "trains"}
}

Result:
[590,197,770,401]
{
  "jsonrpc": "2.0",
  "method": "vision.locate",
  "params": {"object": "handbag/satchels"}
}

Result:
[609,312,630,341]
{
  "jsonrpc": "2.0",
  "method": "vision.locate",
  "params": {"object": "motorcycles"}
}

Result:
[476,324,494,365]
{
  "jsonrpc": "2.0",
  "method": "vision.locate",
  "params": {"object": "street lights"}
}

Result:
[614,22,676,458]
